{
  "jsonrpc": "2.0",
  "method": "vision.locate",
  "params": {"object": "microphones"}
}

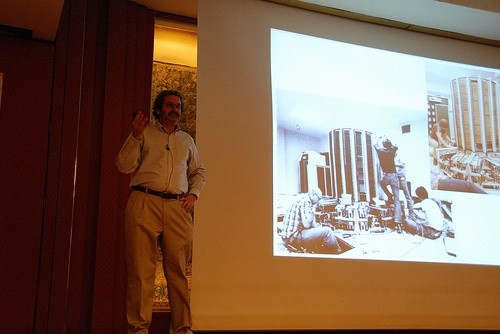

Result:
[166,144,170,151]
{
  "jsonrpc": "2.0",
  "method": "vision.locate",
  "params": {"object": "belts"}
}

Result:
[131,186,184,198]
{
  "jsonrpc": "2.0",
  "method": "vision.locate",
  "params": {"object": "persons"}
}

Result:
[114,90,207,334]
[402,186,443,240]
[393,142,415,219]
[281,187,341,255]
[429,118,456,160]
[372,134,402,233]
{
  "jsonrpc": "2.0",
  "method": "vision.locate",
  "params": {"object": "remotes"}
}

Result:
[132,112,137,118]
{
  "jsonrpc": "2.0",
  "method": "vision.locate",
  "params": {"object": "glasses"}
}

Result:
[162,102,182,110]
[315,194,321,201]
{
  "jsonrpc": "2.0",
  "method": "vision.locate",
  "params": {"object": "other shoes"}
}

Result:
[397,223,402,233]
[387,193,394,204]
